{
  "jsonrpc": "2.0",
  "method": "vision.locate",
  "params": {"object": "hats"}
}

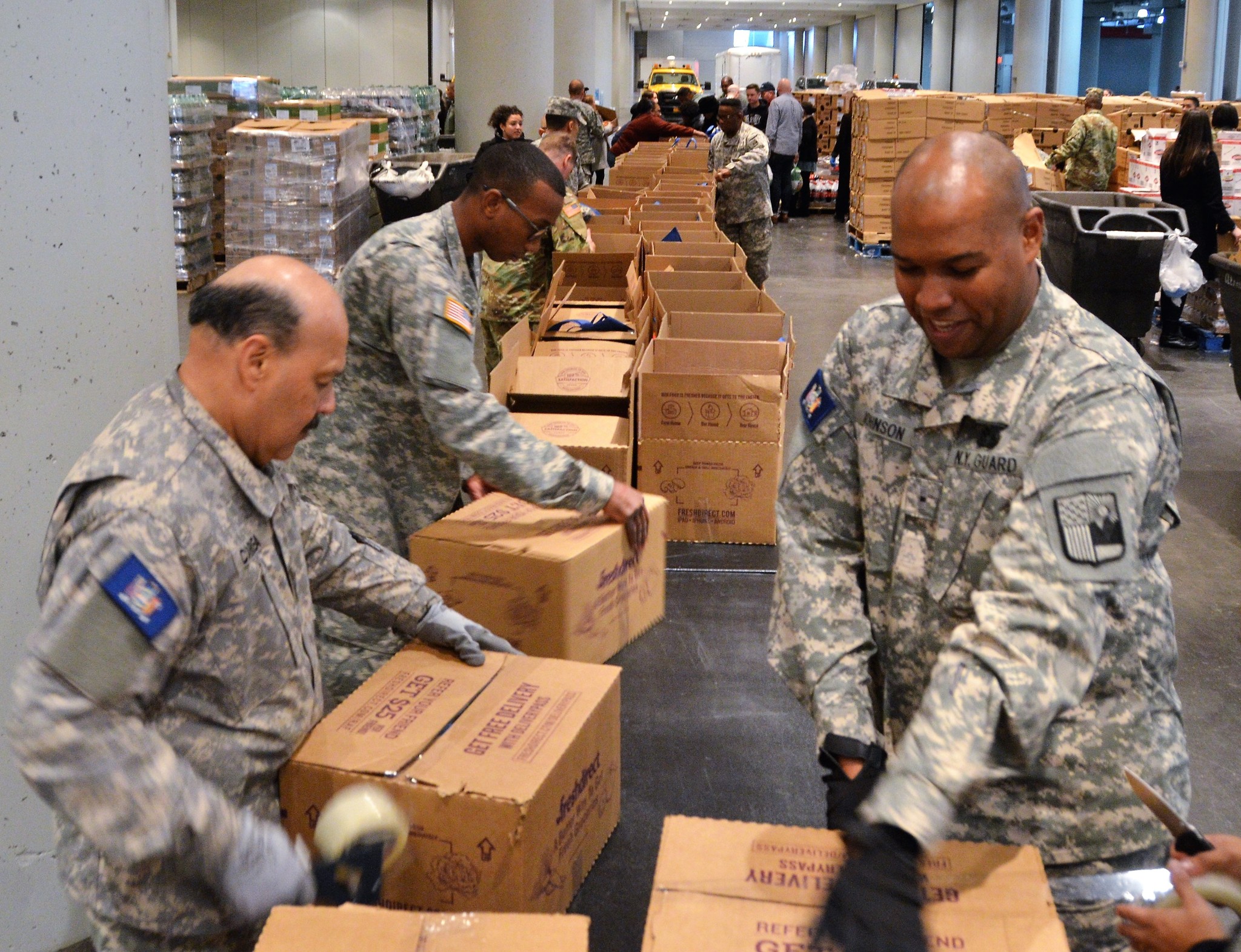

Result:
[759,82,775,92]
[545,96,587,127]
[1084,86,1103,100]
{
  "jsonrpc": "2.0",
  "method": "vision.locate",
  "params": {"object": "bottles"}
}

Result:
[810,178,839,203]
[281,84,441,153]
[169,92,216,279]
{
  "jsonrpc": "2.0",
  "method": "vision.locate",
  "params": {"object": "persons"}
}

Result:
[829,95,859,221]
[1159,108,1241,350]
[476,104,536,163]
[609,73,819,223]
[527,97,589,194]
[1114,833,1241,952]
[10,252,528,952]
[1046,87,1118,193]
[553,82,614,186]
[711,98,776,292]
[1175,96,1200,131]
[1210,104,1239,140]
[436,79,457,135]
[479,130,596,381]
[706,98,773,289]
[281,142,650,709]
[772,131,1190,952]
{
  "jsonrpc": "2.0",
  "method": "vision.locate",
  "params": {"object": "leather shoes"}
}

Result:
[1159,331,1198,349]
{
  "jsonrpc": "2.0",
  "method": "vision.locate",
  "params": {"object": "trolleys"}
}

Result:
[1206,249,1241,401]
[1031,189,1190,358]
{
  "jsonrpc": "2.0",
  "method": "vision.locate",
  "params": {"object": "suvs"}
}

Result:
[637,65,711,117]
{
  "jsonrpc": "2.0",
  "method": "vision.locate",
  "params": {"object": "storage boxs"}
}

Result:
[166,75,1241,952]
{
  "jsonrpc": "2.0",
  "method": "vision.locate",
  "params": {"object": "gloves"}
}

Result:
[222,807,315,925]
[604,123,613,134]
[817,733,888,829]
[409,599,527,667]
[809,821,930,952]
[830,156,836,168]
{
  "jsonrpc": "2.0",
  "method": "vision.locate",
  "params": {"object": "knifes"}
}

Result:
[1123,767,1215,857]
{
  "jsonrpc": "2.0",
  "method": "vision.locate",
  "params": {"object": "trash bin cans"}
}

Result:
[1208,252,1241,400]
[369,153,475,225]
[437,134,455,150]
[1031,191,1190,357]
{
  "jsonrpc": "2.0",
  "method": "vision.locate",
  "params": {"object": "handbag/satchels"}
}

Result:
[790,161,803,195]
[1158,228,1207,299]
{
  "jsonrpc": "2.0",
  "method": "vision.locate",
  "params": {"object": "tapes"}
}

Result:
[1159,871,1240,916]
[314,783,408,874]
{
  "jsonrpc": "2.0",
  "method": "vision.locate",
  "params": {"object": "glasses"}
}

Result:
[716,110,740,121]
[483,184,549,242]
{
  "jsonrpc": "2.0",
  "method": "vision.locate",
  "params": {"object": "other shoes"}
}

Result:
[834,212,845,223]
[777,213,788,223]
[771,215,777,224]
